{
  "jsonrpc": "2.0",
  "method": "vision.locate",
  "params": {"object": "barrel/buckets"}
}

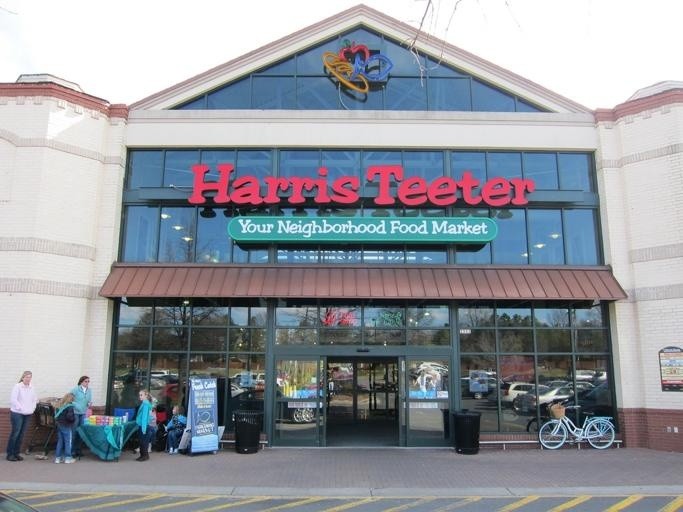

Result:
[114,407,135,422]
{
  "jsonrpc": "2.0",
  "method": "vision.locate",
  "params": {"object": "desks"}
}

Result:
[71,418,137,459]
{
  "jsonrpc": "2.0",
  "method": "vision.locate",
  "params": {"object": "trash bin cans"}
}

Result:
[453,409,481,454]
[233,410,262,454]
[440,408,470,438]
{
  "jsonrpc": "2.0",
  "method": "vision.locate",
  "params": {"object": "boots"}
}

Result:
[135,448,150,461]
[169,447,178,455]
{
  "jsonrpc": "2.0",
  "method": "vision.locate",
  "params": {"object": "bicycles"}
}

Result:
[538,398,615,449]
[526,403,597,434]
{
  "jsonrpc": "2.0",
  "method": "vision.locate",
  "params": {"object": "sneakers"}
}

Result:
[54,456,64,463]
[7,454,24,461]
[64,456,77,464]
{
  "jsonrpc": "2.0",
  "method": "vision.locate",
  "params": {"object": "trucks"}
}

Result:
[383,363,398,391]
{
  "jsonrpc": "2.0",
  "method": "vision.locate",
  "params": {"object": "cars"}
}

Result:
[328,366,353,394]
[550,379,612,425]
[409,361,607,411]
[112,368,322,424]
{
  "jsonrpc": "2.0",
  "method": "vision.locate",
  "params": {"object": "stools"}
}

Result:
[164,428,186,453]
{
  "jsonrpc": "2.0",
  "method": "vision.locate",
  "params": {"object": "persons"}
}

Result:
[67,376,93,458]
[134,394,160,453]
[53,393,77,464]
[166,404,187,455]
[6,370,40,461]
[120,375,139,407]
[135,388,152,461]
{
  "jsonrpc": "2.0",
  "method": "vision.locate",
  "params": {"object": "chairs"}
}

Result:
[111,406,136,421]
